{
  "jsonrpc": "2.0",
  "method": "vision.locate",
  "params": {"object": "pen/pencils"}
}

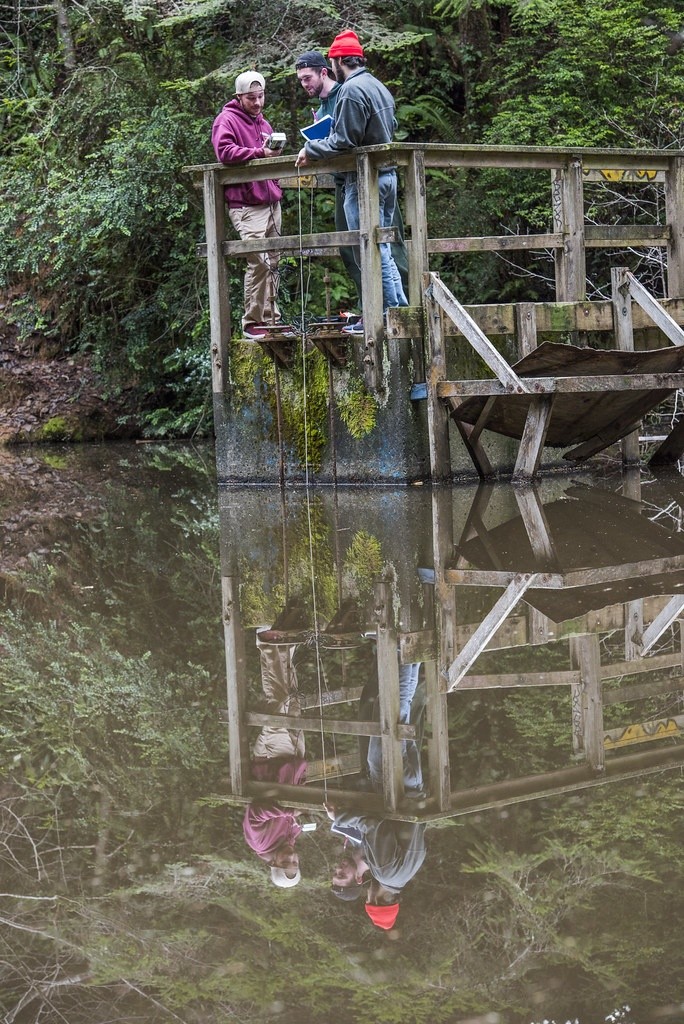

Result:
[310,107,319,123]
[343,838,349,850]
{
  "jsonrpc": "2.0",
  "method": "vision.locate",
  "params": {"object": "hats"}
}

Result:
[295,50,334,76]
[364,901,399,930]
[328,29,363,56]
[330,884,363,901]
[269,865,301,887]
[232,70,266,96]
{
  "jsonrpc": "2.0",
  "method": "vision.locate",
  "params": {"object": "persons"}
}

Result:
[295,52,383,322]
[295,29,413,337]
[237,625,430,933]
[211,69,297,340]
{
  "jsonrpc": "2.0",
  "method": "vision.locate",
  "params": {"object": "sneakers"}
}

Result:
[243,322,269,339]
[342,323,364,334]
[267,324,295,337]
